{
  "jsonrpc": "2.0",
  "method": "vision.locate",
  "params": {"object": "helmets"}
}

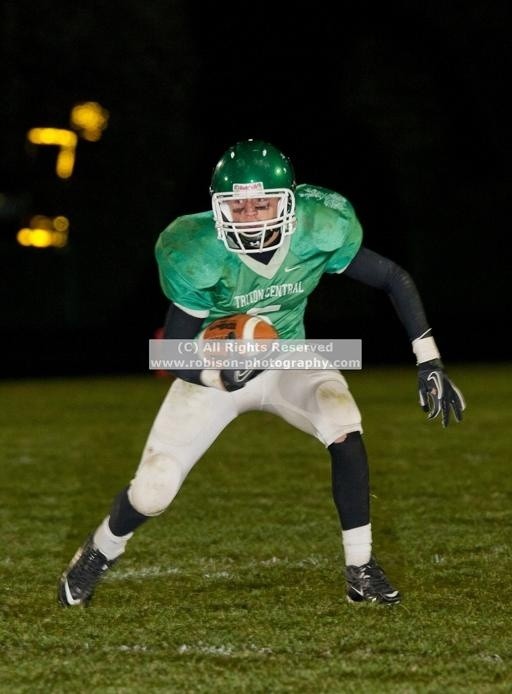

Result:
[209,137,297,254]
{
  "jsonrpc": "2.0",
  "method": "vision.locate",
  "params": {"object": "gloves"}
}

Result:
[416,368,466,428]
[219,346,280,392]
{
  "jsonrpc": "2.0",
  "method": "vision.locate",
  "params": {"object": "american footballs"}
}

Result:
[197,316,281,371]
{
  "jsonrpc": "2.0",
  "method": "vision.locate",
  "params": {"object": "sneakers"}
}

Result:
[57,519,118,608]
[344,552,402,607]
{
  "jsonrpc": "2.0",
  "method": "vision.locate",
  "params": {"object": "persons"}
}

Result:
[58,139,467,609]
